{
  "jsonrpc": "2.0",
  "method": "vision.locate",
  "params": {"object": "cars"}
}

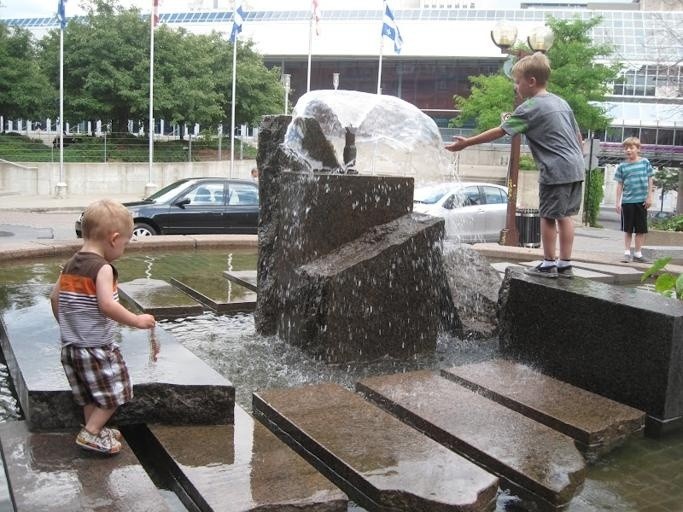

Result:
[73,176,261,244]
[413,182,508,243]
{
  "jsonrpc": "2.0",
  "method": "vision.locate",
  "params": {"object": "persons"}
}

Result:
[246,168,258,184]
[49,198,157,456]
[442,49,586,280]
[610,135,654,263]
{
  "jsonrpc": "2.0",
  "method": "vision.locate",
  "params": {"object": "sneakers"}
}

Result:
[73,426,122,454]
[632,255,651,264]
[554,263,575,278]
[521,262,558,279]
[78,423,122,440]
[620,255,630,263]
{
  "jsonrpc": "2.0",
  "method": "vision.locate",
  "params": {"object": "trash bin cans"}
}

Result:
[515,209,540,247]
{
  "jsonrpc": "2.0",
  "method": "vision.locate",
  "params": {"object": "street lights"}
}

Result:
[332,73,340,89]
[488,18,554,247]
[282,74,291,115]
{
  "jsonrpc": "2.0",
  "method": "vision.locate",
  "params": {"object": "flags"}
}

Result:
[380,1,404,55]
[152,0,163,27]
[305,0,322,39]
[225,0,250,45]
[53,0,68,30]
